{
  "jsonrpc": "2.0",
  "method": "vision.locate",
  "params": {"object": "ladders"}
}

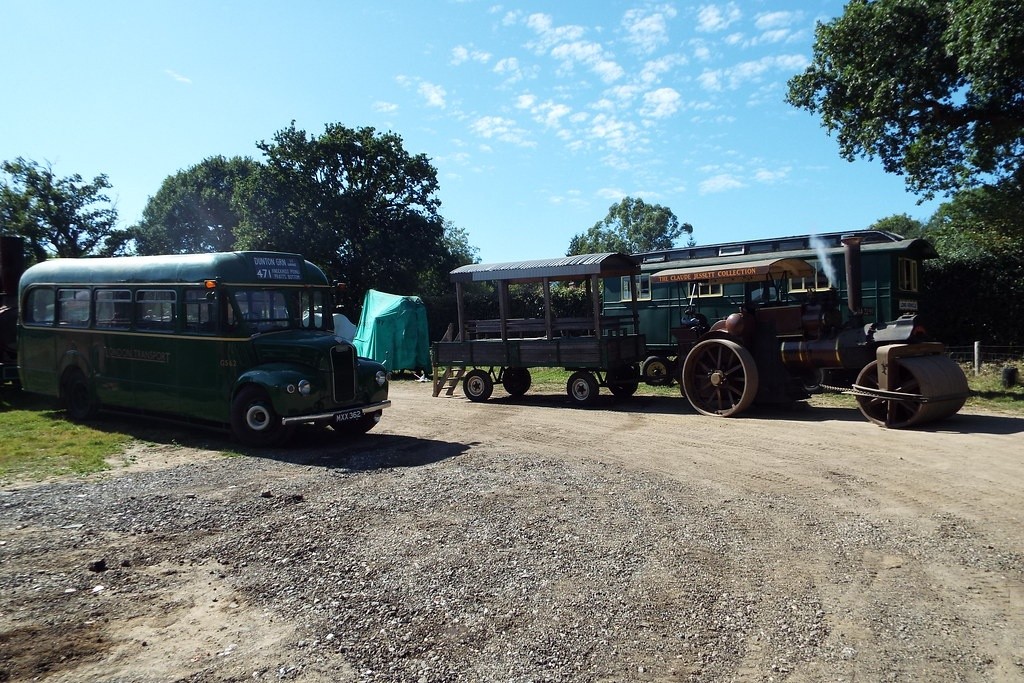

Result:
[430,325,467,397]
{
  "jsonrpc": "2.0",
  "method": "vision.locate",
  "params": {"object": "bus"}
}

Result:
[16,249,392,450]
